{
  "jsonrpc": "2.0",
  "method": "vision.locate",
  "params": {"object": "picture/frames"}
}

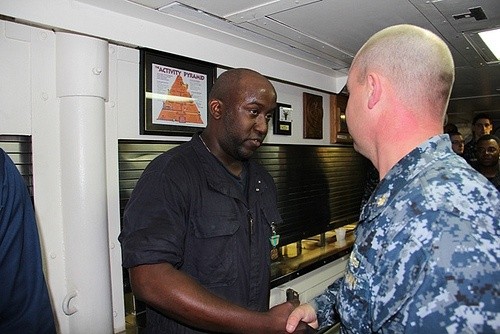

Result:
[139,47,215,137]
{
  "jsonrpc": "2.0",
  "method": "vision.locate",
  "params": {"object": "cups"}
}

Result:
[335,229,346,240]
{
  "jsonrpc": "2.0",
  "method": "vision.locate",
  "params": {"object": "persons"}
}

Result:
[0,148,58,334]
[285,24,500,334]
[117,68,318,334]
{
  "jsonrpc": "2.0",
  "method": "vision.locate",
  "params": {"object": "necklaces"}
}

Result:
[199,135,211,151]
